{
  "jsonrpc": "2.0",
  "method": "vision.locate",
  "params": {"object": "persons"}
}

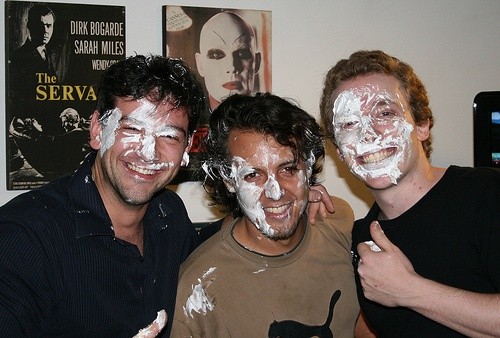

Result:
[9,5,68,118]
[170,92,362,338]
[194,11,262,124]
[0,55,334,338]
[319,51,499,338]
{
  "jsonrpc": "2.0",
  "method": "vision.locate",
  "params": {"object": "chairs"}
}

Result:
[473,90,500,167]
